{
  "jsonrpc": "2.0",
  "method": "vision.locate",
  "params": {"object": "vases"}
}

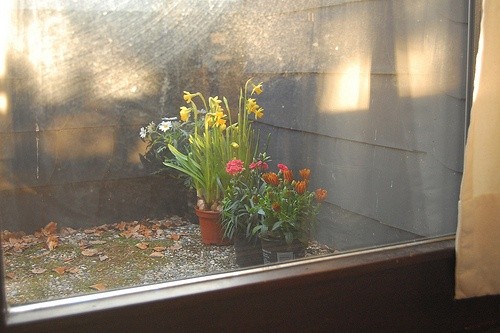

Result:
[193,204,308,270]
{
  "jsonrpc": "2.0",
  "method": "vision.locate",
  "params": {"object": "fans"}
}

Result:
[140,75,330,243]
